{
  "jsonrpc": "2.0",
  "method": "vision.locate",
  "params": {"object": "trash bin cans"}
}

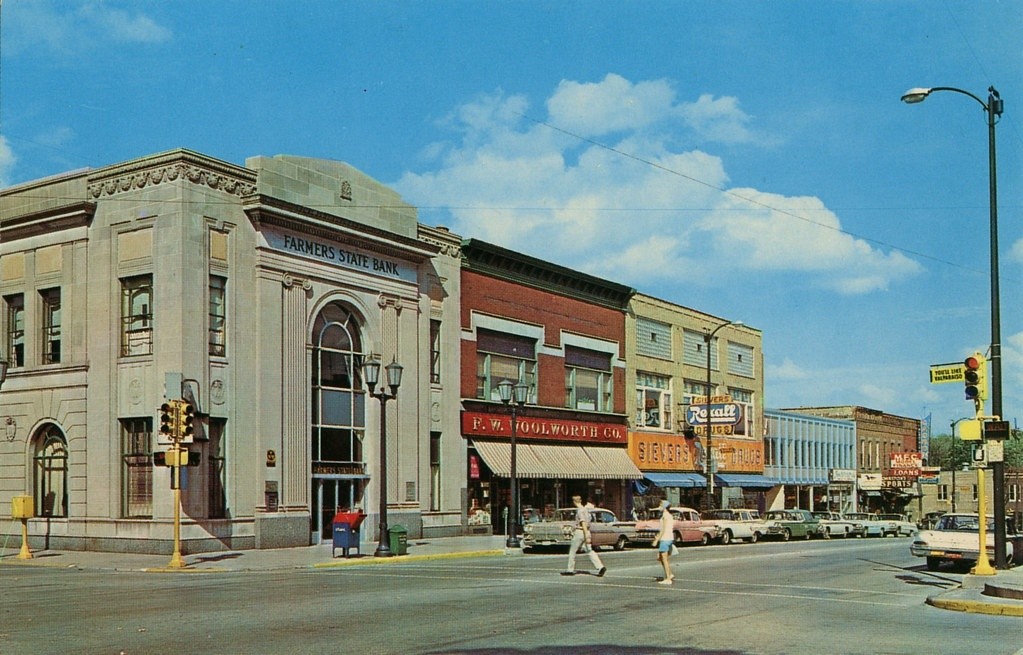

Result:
[387,525,408,555]
[333,509,367,548]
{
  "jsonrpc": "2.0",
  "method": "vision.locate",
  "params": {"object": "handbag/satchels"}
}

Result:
[669,544,679,556]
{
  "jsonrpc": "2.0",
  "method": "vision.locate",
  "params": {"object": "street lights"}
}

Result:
[951,417,975,516]
[497,372,530,549]
[362,351,404,557]
[704,321,744,509]
[901,84,1012,572]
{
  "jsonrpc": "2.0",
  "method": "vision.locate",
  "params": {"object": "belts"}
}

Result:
[576,527,590,530]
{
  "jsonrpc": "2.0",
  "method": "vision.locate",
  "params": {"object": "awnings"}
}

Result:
[469,437,646,480]
[713,474,774,488]
[634,473,718,495]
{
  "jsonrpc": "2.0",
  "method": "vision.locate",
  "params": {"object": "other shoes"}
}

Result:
[560,571,574,575]
[597,567,606,576]
[657,574,675,585]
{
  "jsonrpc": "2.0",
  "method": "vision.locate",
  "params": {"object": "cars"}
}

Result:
[843,512,886,539]
[908,512,1016,570]
[700,509,769,544]
[809,510,854,538]
[760,509,820,541]
[523,507,637,551]
[880,513,918,538]
[634,506,721,546]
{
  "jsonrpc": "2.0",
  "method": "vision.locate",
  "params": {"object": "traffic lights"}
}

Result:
[177,401,195,438]
[154,451,176,466]
[964,351,987,403]
[160,401,177,438]
[180,450,201,466]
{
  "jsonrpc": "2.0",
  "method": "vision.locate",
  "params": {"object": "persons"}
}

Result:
[652,499,678,586]
[561,494,607,576]
[872,504,898,520]
